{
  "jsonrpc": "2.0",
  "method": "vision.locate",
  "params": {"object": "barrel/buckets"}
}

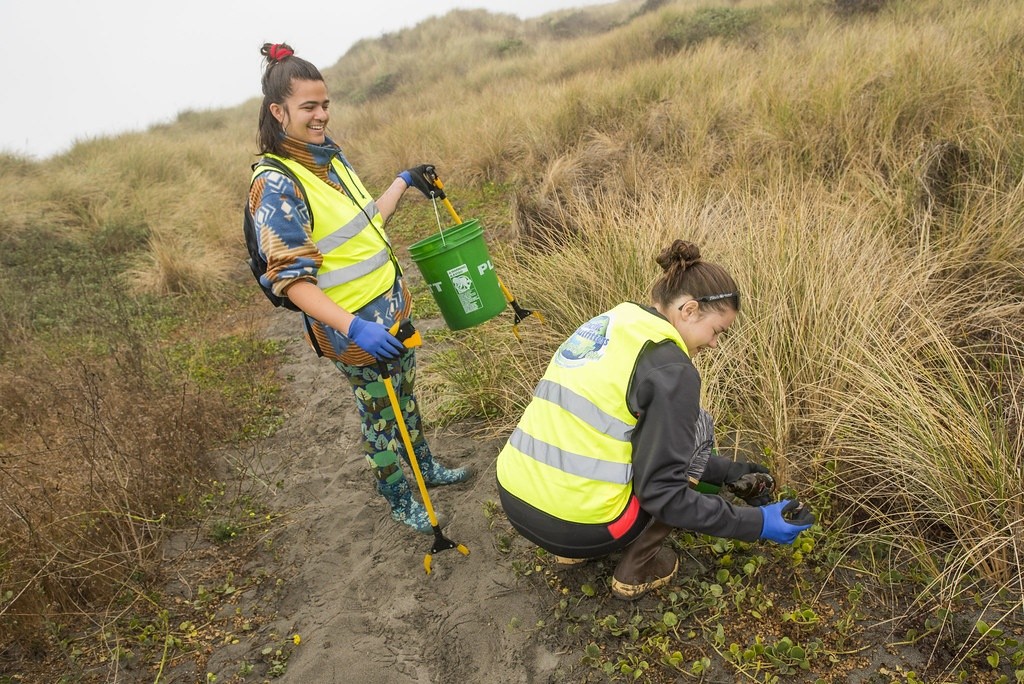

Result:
[407,187,508,332]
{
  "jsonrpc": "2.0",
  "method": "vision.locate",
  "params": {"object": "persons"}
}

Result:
[243,42,476,535]
[494,238,814,602]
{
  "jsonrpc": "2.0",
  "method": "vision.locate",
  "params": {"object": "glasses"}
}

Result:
[677,291,741,311]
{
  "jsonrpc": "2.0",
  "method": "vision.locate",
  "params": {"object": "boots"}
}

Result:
[555,555,595,565]
[611,476,699,600]
[397,440,475,487]
[377,474,449,534]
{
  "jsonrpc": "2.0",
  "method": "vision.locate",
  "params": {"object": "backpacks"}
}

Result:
[243,157,314,312]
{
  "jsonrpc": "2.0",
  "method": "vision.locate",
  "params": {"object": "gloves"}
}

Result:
[347,316,409,364]
[759,499,816,543]
[725,461,774,506]
[396,165,443,199]
